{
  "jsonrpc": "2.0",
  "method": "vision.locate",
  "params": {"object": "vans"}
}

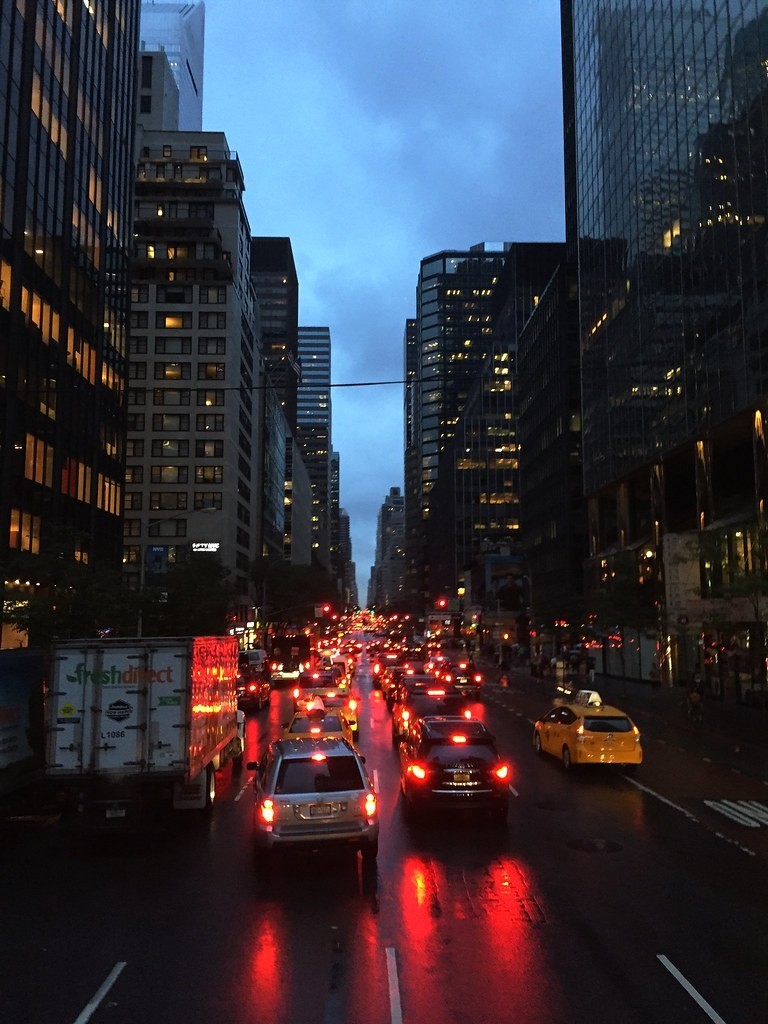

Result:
[239,650,270,675]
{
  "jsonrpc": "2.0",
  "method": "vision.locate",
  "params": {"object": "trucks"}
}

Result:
[268,633,311,681]
[44,634,248,822]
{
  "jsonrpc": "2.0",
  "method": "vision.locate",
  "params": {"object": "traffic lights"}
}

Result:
[322,602,331,614]
[438,599,448,609]
[503,632,510,642]
[331,614,338,621]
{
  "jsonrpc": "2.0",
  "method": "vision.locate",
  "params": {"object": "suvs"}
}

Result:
[245,735,382,860]
[394,716,511,821]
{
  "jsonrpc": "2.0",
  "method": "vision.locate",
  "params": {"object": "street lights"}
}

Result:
[262,558,292,621]
[137,506,216,637]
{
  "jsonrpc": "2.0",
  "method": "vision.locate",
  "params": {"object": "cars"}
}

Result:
[533,690,643,770]
[281,696,355,748]
[237,608,485,735]
[551,651,581,669]
